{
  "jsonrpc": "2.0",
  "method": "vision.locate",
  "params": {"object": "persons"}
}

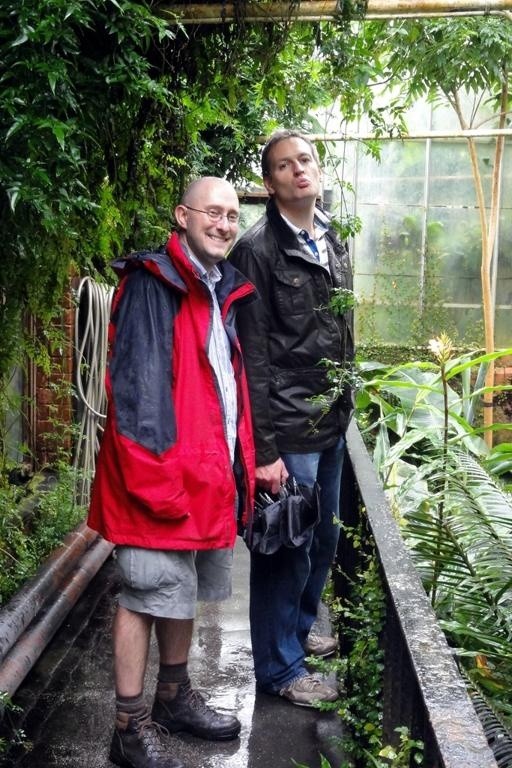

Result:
[224,129,354,709]
[85,174,259,767]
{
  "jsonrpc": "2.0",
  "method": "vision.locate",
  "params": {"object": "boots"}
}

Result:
[109,710,184,767]
[150,681,240,739]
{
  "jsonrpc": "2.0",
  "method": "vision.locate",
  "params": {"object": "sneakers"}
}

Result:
[276,674,339,707]
[304,634,337,657]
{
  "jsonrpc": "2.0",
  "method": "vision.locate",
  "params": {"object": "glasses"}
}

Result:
[183,204,240,223]
